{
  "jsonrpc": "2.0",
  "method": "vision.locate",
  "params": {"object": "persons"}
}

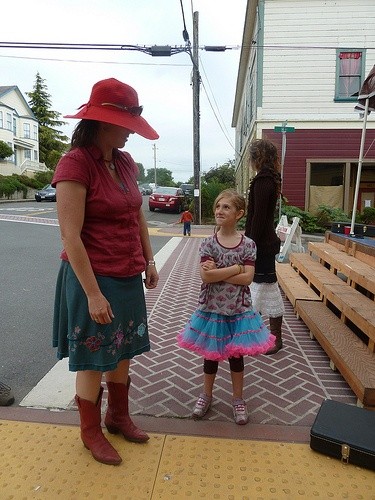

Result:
[50,78,159,464]
[181,205,194,236]
[244,139,285,355]
[176,187,277,425]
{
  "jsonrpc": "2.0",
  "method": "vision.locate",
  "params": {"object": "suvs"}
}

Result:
[179,183,194,202]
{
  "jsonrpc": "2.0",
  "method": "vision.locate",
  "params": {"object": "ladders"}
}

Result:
[275,215,304,262]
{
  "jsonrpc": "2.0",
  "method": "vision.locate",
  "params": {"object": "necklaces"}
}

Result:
[104,159,116,170]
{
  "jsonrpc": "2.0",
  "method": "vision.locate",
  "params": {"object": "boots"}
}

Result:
[264,316,283,355]
[75,385,122,465]
[104,375,150,442]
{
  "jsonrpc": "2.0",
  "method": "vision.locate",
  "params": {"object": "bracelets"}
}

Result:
[234,264,242,274]
[146,261,155,266]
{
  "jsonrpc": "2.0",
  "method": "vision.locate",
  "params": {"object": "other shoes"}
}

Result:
[0,382,15,405]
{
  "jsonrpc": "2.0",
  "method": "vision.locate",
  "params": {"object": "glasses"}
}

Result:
[102,102,144,116]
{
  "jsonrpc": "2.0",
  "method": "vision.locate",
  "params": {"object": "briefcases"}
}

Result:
[309,399,375,471]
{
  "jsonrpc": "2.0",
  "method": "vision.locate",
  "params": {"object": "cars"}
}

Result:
[148,187,186,214]
[35,184,56,202]
[137,184,152,196]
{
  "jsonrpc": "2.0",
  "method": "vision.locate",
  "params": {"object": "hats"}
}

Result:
[63,78,160,140]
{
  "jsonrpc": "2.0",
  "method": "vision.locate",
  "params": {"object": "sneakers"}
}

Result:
[232,398,248,424]
[193,393,212,417]
